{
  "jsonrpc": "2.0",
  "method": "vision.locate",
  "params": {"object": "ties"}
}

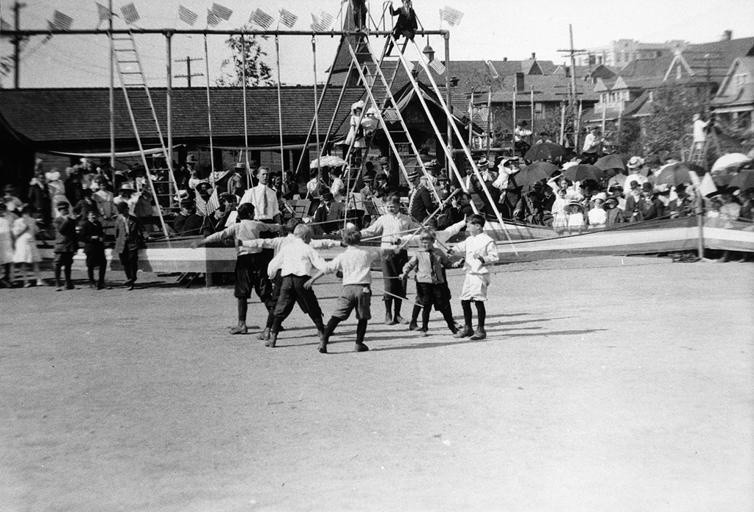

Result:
[428,250,434,276]
[263,186,268,215]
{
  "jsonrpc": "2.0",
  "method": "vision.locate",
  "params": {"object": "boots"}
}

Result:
[393,312,409,324]
[265,329,277,347]
[318,323,335,336]
[452,324,474,338]
[470,323,486,340]
[256,327,270,341]
[230,320,247,334]
[384,312,395,325]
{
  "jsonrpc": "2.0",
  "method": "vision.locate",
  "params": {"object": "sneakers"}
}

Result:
[318,342,329,353]
[355,344,369,351]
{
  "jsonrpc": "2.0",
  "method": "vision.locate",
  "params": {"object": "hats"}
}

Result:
[608,183,623,192]
[186,155,198,163]
[234,162,245,169]
[476,158,489,167]
[406,171,420,180]
[349,100,365,116]
[589,192,607,202]
[642,182,653,192]
[625,156,644,169]
[673,182,687,193]
[629,181,641,189]
[563,199,584,211]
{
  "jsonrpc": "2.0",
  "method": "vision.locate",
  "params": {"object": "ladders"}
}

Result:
[345,34,444,211]
[339,34,409,195]
[468,85,492,157]
[560,99,582,154]
[688,127,710,166]
[107,29,182,248]
[513,85,534,157]
[601,100,624,153]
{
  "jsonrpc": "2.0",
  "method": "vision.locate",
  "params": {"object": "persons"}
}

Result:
[582,125,604,166]
[467,156,753,265]
[234,222,346,341]
[301,227,402,353]
[692,114,709,159]
[342,99,369,158]
[171,166,464,247]
[361,193,432,325]
[190,203,285,335]
[265,225,330,350]
[2,163,146,290]
[514,119,552,165]
[449,213,501,341]
[398,229,469,335]
[382,1,419,57]
